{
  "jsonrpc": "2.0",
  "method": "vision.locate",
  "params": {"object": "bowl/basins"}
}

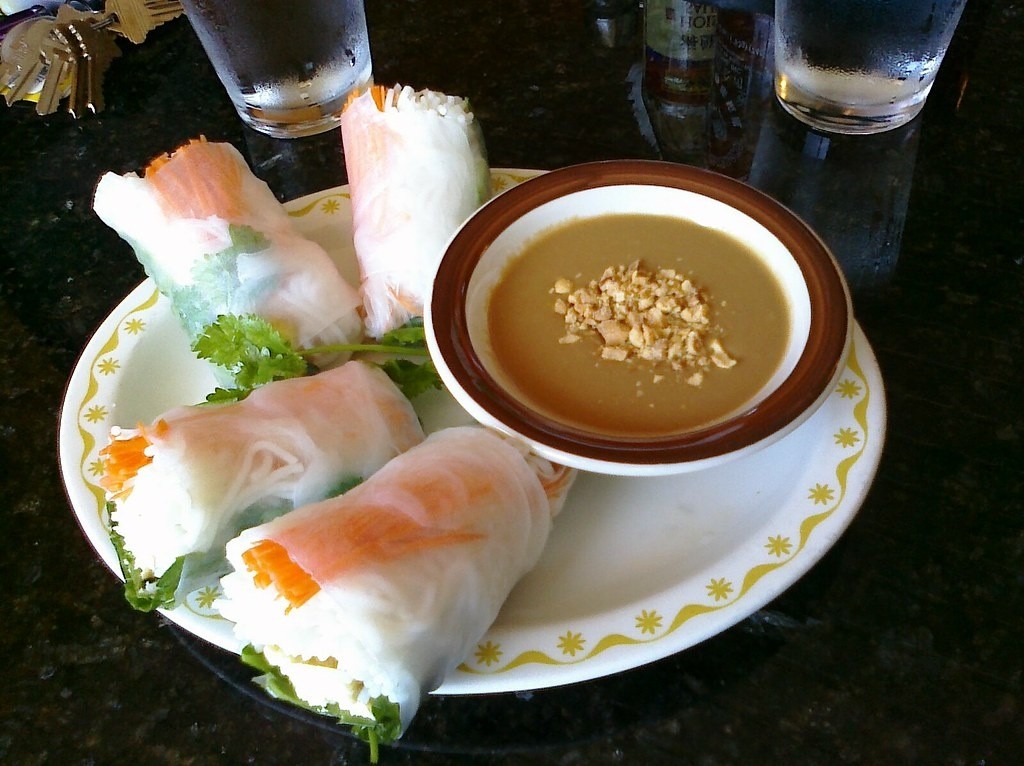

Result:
[416,155,854,480]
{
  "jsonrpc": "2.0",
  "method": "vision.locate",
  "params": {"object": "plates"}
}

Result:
[59,164,887,698]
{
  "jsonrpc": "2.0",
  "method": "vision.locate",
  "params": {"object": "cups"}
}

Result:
[771,1,970,137]
[178,0,374,141]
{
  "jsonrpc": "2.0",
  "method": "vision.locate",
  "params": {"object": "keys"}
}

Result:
[0,0,187,120]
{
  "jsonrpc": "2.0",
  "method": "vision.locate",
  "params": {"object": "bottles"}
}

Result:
[639,0,774,191]
